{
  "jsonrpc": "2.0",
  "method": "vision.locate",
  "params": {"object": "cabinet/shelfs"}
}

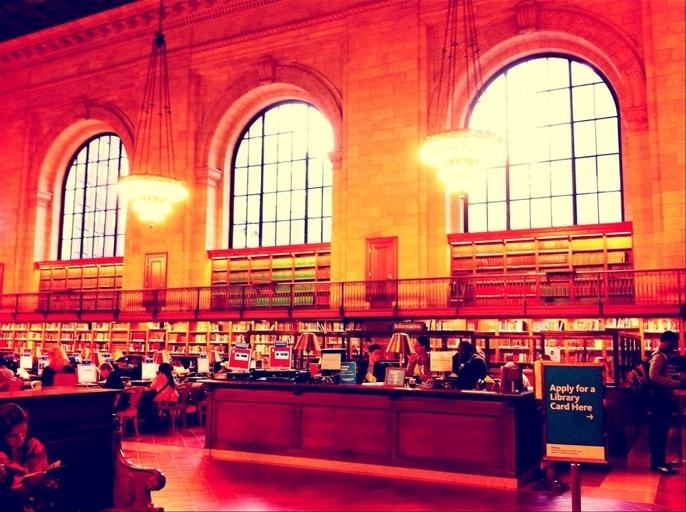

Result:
[0,316,685,385]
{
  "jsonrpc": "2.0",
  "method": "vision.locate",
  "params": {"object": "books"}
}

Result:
[20,460,65,483]
[0,317,681,370]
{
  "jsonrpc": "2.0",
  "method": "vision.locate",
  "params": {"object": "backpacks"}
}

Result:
[627,353,667,397]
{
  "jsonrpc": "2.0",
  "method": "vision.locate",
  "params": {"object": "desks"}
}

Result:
[0,373,540,512]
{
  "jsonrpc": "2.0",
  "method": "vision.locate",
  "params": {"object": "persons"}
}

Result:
[645,331,681,475]
[357,343,386,383]
[0,402,62,512]
[0,345,185,412]
[452,341,488,390]
[405,335,445,380]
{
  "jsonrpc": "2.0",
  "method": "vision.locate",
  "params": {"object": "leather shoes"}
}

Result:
[651,463,677,474]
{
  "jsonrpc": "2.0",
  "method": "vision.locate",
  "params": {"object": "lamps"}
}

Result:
[115,0,193,226]
[418,1,503,198]
